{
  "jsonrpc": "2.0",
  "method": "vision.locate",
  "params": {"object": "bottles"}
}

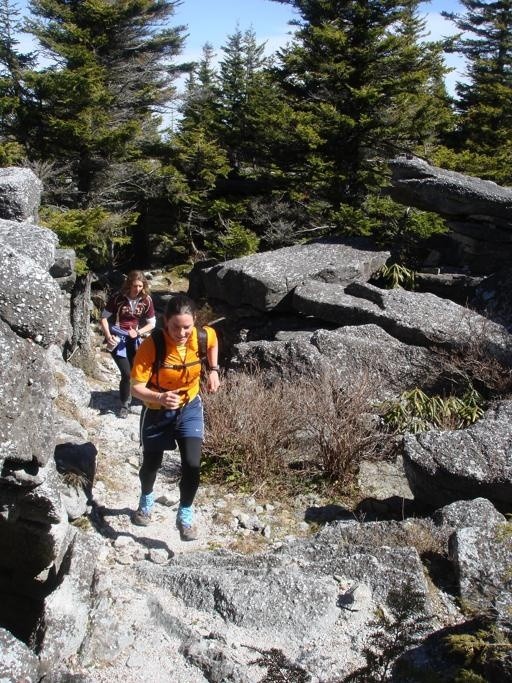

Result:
[105,335,121,353]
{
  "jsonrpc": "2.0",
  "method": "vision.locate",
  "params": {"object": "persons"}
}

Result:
[131,296,221,542]
[100,269,157,418]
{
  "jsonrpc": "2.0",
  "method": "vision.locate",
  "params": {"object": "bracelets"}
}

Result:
[209,367,220,372]
[137,330,140,335]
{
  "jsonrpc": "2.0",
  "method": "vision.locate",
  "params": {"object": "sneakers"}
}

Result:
[117,400,128,418]
[133,492,154,526]
[175,503,199,541]
